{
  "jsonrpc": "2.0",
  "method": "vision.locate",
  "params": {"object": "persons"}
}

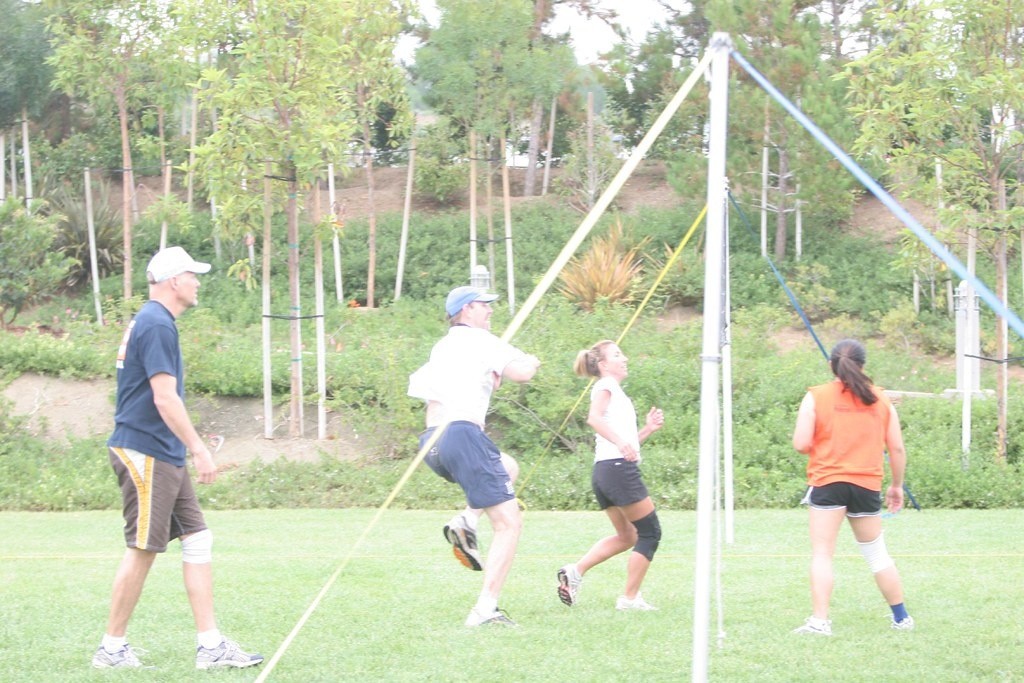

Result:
[557,340,666,613]
[93,245,265,672]
[792,338,915,633]
[407,286,540,628]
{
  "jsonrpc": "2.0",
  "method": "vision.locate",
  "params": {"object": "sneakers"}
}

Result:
[443,514,484,571]
[91,641,155,672]
[196,638,264,669]
[465,607,521,630]
[791,615,833,635]
[883,614,914,631]
[615,591,659,612]
[558,563,582,606]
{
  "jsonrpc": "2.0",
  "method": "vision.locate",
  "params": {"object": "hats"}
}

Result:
[147,246,212,283]
[446,286,500,317]
